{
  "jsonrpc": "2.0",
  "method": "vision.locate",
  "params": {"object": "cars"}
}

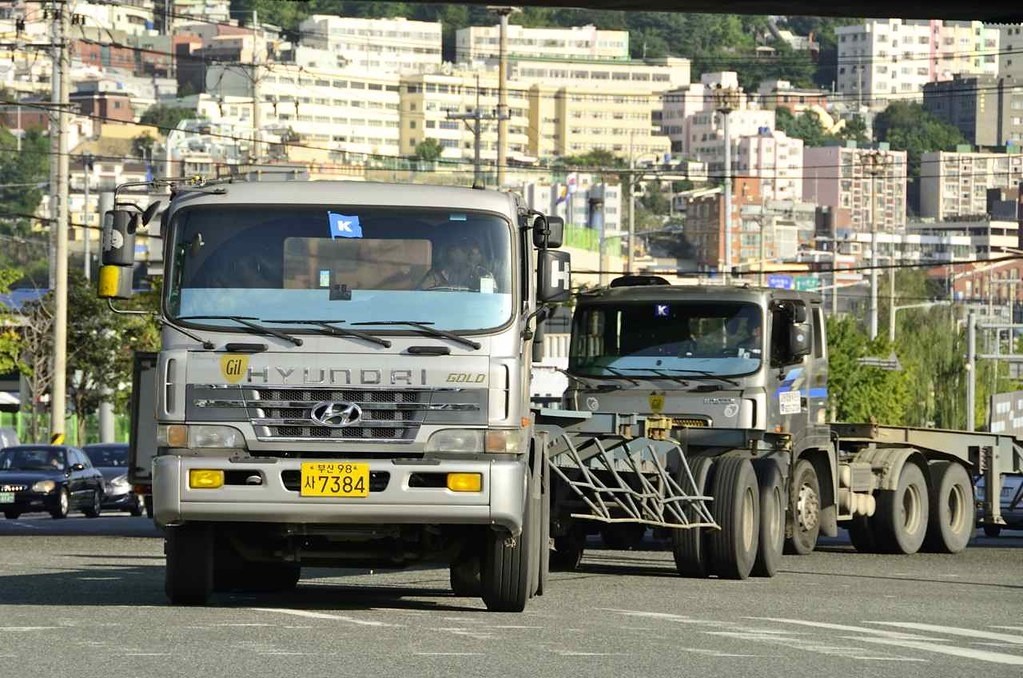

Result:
[82,443,144,516]
[0,444,105,520]
[973,471,1023,536]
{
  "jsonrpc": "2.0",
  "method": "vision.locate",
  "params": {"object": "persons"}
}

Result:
[48,455,65,470]
[737,315,782,368]
[417,237,498,295]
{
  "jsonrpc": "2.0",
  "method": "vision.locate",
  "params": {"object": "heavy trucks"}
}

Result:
[95,175,638,613]
[550,274,1023,580]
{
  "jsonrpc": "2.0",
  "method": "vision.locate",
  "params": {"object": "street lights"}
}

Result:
[859,151,894,343]
[888,300,952,344]
